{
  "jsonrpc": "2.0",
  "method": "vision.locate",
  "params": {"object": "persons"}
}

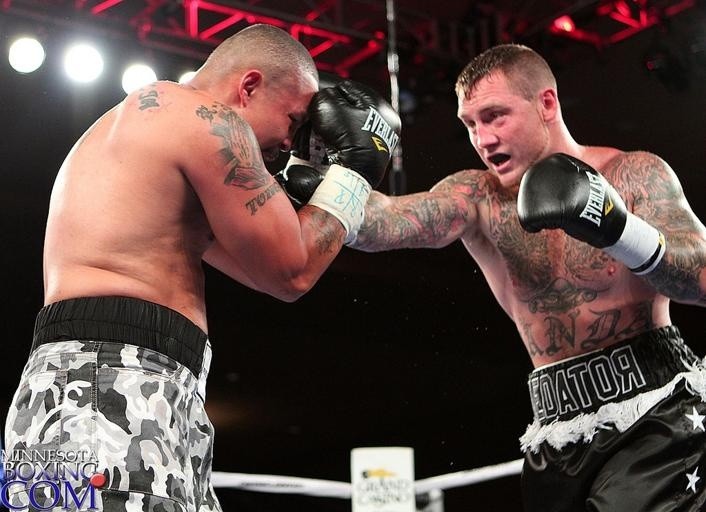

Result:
[4,24,404,512]
[272,44,706,511]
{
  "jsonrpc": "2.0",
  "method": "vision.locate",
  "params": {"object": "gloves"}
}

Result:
[274,77,402,210]
[516,152,627,247]
